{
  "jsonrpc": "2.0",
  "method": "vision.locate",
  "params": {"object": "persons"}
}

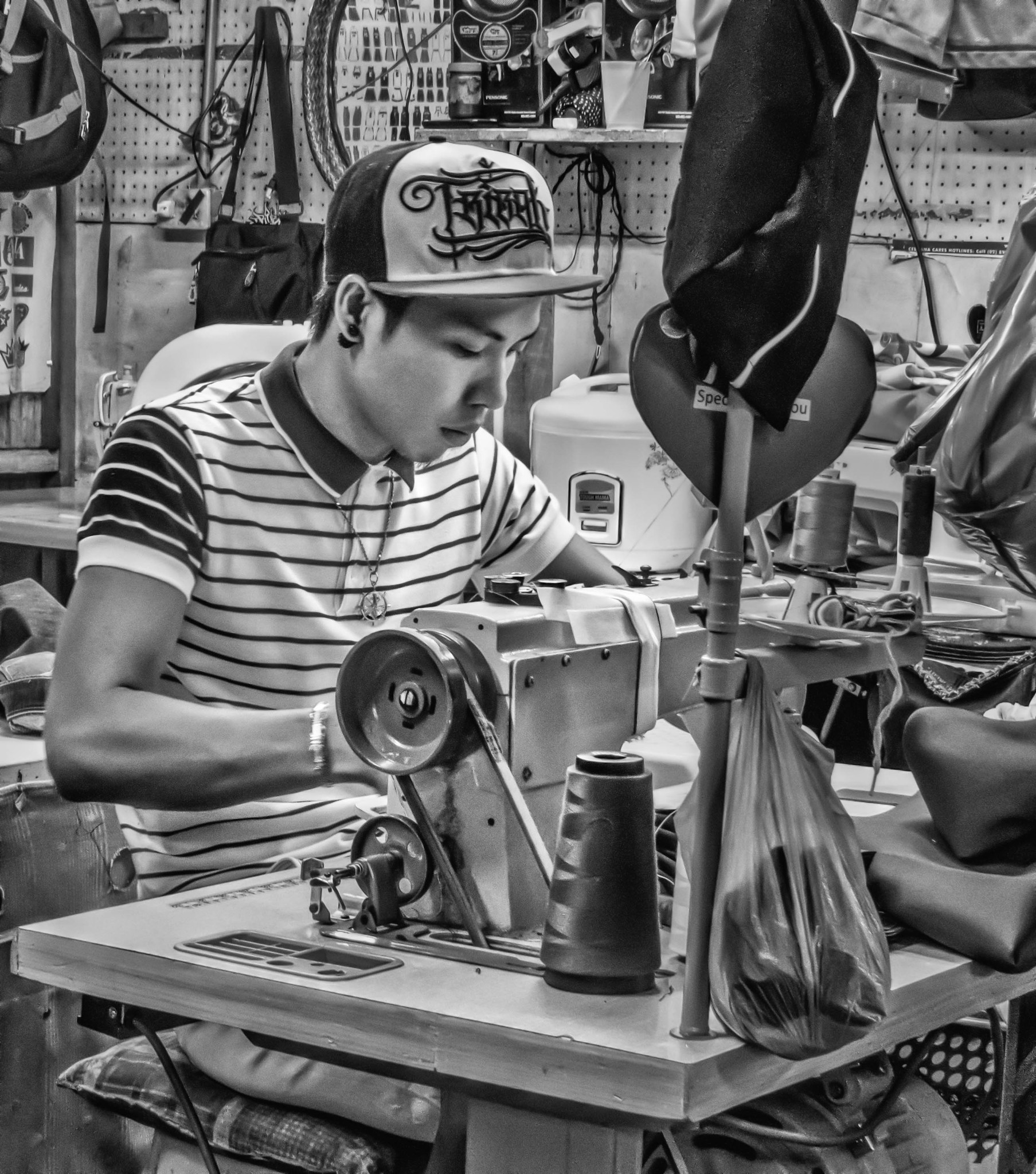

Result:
[41,135,705,1173]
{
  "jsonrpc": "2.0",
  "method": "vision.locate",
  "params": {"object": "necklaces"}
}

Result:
[336,470,396,625]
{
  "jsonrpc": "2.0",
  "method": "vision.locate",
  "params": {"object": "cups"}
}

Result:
[600,59,650,129]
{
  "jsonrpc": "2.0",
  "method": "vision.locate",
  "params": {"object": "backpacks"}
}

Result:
[0,1,109,190]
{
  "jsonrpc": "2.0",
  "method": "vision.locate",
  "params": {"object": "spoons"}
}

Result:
[613,30,672,120]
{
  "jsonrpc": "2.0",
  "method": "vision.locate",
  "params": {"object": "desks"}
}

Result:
[5,751,1035,1174]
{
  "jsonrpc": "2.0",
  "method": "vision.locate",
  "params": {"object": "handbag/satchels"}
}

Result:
[192,212,325,329]
[706,656,894,1059]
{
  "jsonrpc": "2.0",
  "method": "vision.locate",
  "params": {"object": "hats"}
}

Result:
[324,135,607,298]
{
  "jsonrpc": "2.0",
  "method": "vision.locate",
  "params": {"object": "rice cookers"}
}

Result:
[529,370,714,576]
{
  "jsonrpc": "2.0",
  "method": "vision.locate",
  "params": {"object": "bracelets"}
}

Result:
[308,703,334,786]
